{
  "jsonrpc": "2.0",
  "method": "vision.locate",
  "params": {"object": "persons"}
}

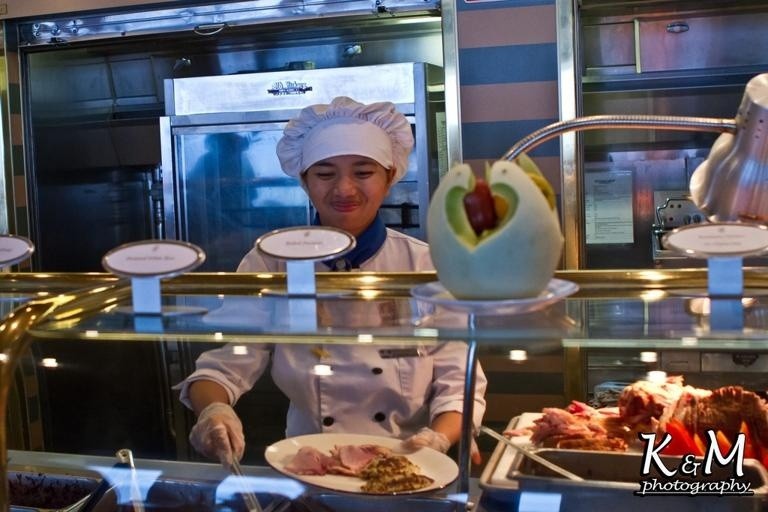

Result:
[172,96,487,470]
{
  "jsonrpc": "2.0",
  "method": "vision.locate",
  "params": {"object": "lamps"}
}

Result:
[501,73,767,227]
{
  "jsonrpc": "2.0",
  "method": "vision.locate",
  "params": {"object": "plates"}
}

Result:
[411,276,581,318]
[262,433,459,496]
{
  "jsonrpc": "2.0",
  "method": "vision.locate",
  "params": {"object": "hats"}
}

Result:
[276,96,414,187]
